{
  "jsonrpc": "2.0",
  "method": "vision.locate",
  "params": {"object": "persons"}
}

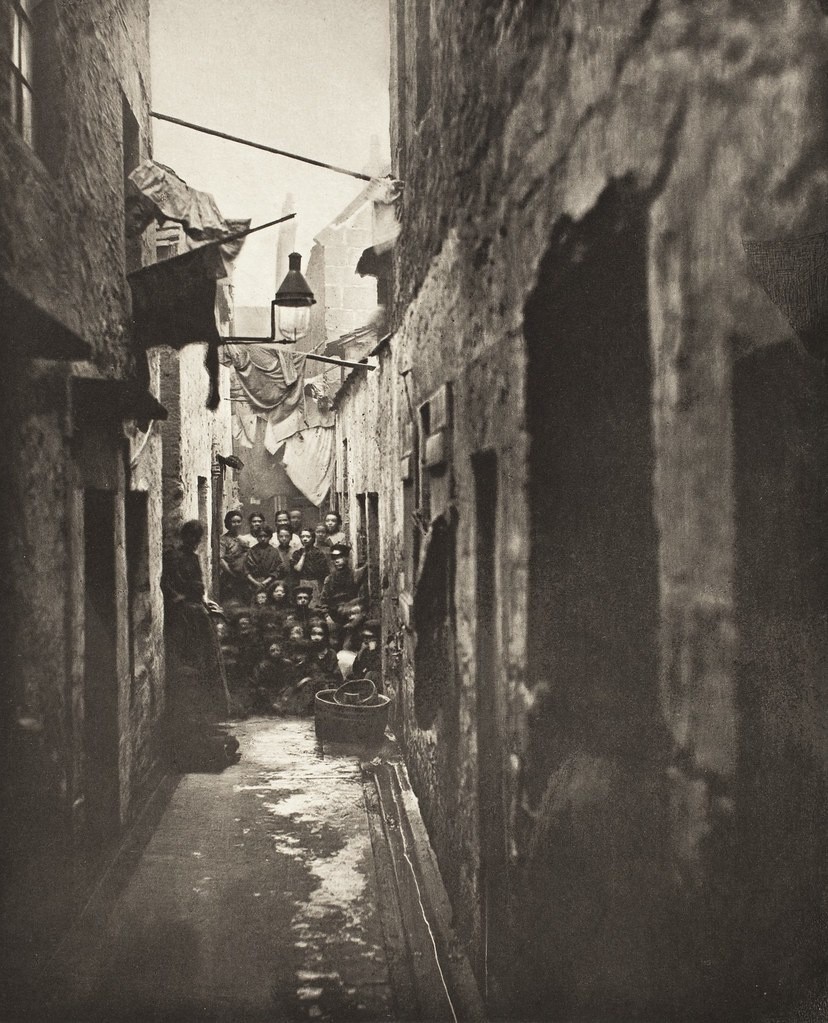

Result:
[160,508,382,735]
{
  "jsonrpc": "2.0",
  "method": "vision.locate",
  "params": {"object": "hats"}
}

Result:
[293,586,313,598]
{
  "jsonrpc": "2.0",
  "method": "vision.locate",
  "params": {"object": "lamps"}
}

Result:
[219,251,317,346]
